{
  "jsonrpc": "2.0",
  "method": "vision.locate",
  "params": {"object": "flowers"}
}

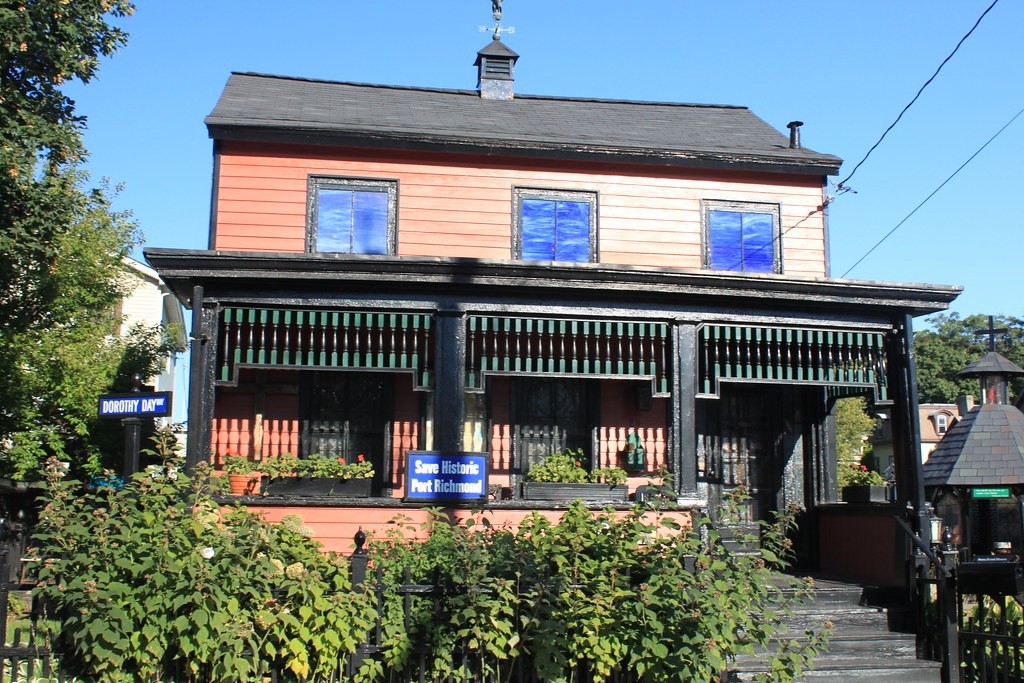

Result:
[223,447,375,483]
[843,464,885,488]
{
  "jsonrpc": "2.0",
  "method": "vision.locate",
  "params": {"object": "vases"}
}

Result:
[229,474,256,495]
[842,487,885,502]
[260,476,372,498]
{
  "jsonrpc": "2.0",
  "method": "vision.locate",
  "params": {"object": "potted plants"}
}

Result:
[519,448,629,503]
